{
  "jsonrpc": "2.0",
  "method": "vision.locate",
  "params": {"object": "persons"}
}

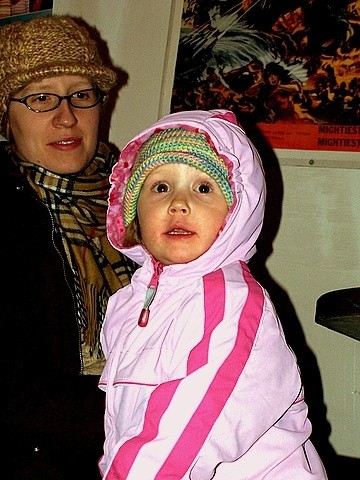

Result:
[98,107,330,480]
[0,12,138,480]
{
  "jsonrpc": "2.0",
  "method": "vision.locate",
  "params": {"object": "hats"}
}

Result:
[121,128,233,225]
[0,15,118,105]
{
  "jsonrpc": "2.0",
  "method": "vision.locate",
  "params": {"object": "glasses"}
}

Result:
[7,88,104,113]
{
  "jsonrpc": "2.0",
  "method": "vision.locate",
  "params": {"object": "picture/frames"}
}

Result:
[158,0,360,172]
